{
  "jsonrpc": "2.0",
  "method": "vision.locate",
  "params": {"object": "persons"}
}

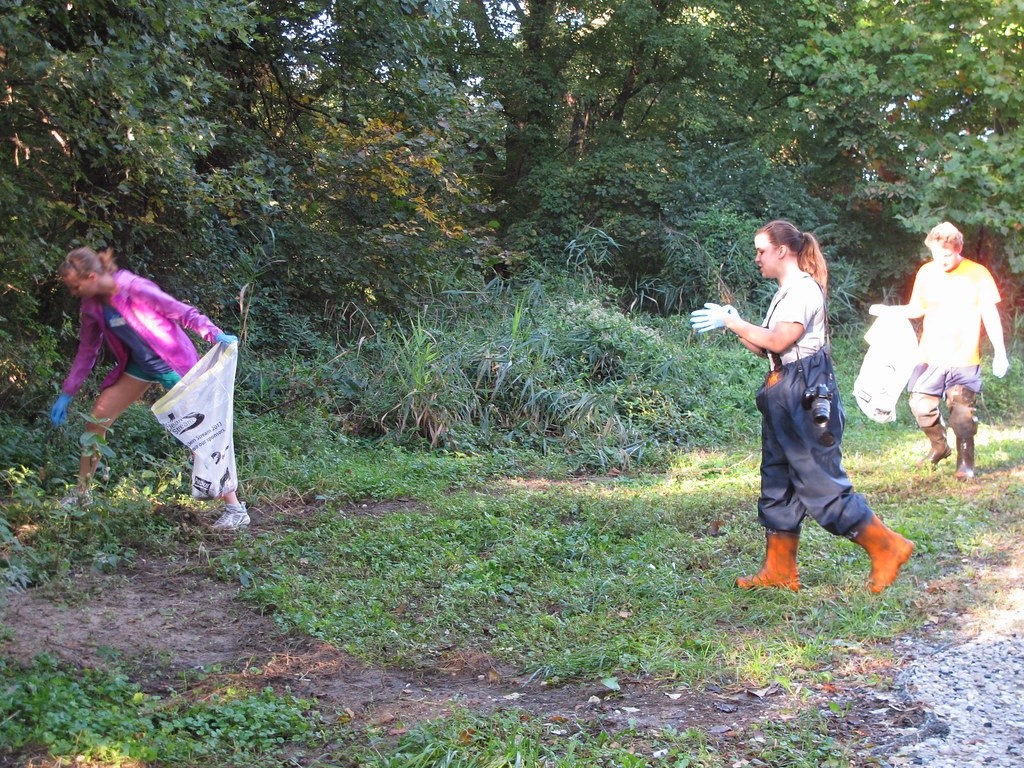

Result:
[688,218,914,596]
[48,247,252,531]
[867,220,1011,480]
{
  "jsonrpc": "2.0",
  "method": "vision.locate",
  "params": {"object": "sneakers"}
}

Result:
[58,492,93,511]
[213,501,251,529]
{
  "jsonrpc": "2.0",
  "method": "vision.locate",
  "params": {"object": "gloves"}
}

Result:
[216,333,238,351]
[51,393,72,426]
[690,302,734,333]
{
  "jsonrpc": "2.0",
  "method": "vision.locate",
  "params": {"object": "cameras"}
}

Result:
[801,385,833,429]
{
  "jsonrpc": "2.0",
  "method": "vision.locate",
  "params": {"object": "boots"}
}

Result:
[915,413,950,470]
[954,436,975,482]
[850,512,915,593]
[737,527,802,592]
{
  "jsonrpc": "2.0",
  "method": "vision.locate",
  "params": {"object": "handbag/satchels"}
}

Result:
[150,340,239,499]
[853,317,918,423]
